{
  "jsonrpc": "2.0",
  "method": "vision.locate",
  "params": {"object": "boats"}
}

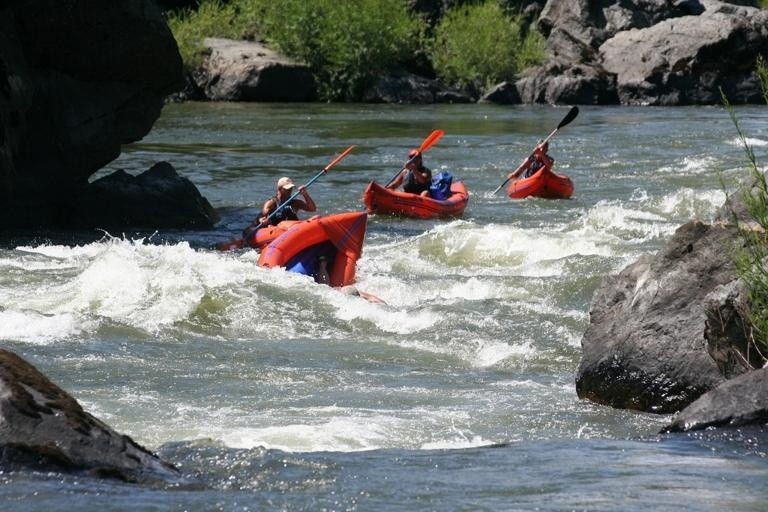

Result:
[213,213,322,251]
[361,178,470,220]
[506,165,575,200]
[258,210,368,287]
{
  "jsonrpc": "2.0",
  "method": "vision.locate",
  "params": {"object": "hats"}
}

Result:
[409,149,422,159]
[278,177,296,190]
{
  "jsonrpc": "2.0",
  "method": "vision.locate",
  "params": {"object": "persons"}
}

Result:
[386,150,432,198]
[507,139,554,180]
[253,177,316,228]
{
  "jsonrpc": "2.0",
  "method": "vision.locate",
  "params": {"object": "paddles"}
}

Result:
[218,146,354,252]
[384,129,445,187]
[493,105,579,195]
